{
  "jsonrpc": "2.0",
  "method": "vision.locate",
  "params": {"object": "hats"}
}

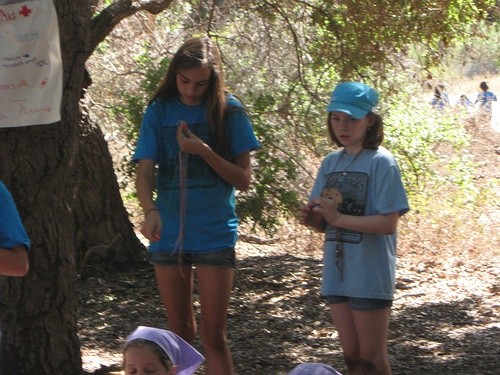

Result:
[327,82,379,119]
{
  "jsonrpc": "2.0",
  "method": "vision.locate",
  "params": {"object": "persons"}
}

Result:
[294,81,411,375]
[0,181,29,276]
[124,326,204,375]
[432,81,498,123]
[133,37,260,374]
